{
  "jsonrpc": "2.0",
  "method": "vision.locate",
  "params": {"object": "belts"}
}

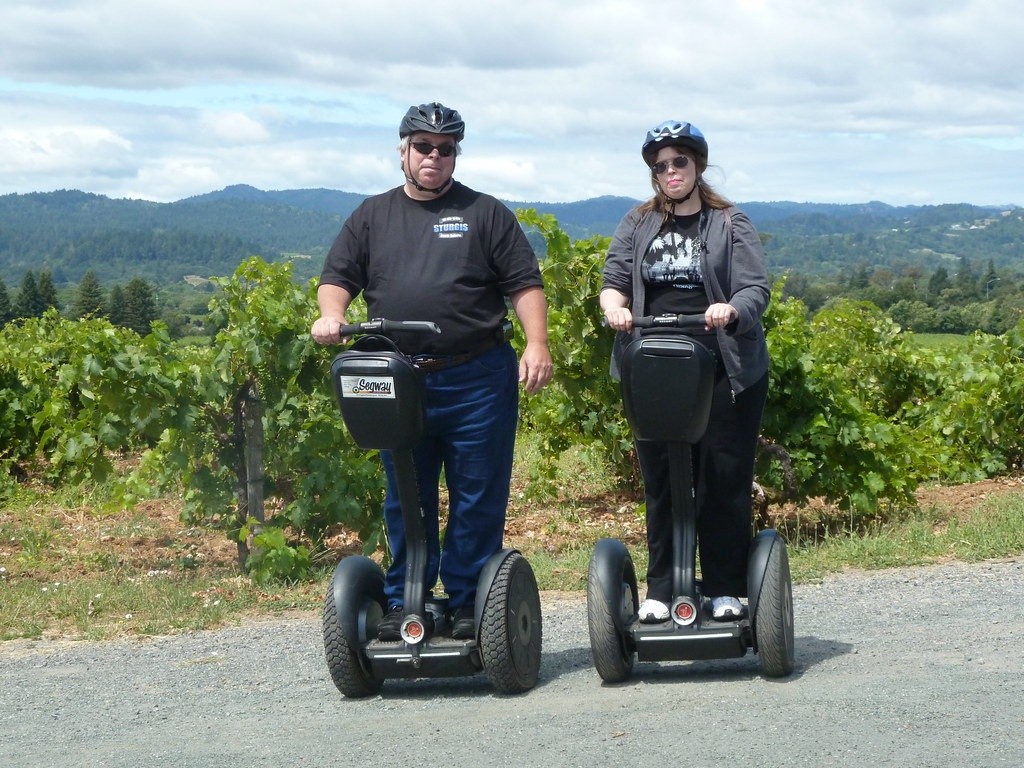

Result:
[406,338,500,374]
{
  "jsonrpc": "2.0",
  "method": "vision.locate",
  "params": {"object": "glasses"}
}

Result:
[406,138,456,157]
[651,155,688,174]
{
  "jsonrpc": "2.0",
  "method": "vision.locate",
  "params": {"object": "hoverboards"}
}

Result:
[320,318,544,702]
[585,313,798,686]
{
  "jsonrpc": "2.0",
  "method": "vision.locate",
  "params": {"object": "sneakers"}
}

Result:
[451,605,477,637]
[377,605,407,640]
[711,595,744,619]
[638,599,670,623]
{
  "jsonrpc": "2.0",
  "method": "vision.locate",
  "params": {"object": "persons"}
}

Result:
[309,103,555,644]
[596,119,774,624]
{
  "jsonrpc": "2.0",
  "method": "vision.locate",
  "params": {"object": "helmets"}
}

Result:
[399,102,465,137]
[642,119,709,158]
